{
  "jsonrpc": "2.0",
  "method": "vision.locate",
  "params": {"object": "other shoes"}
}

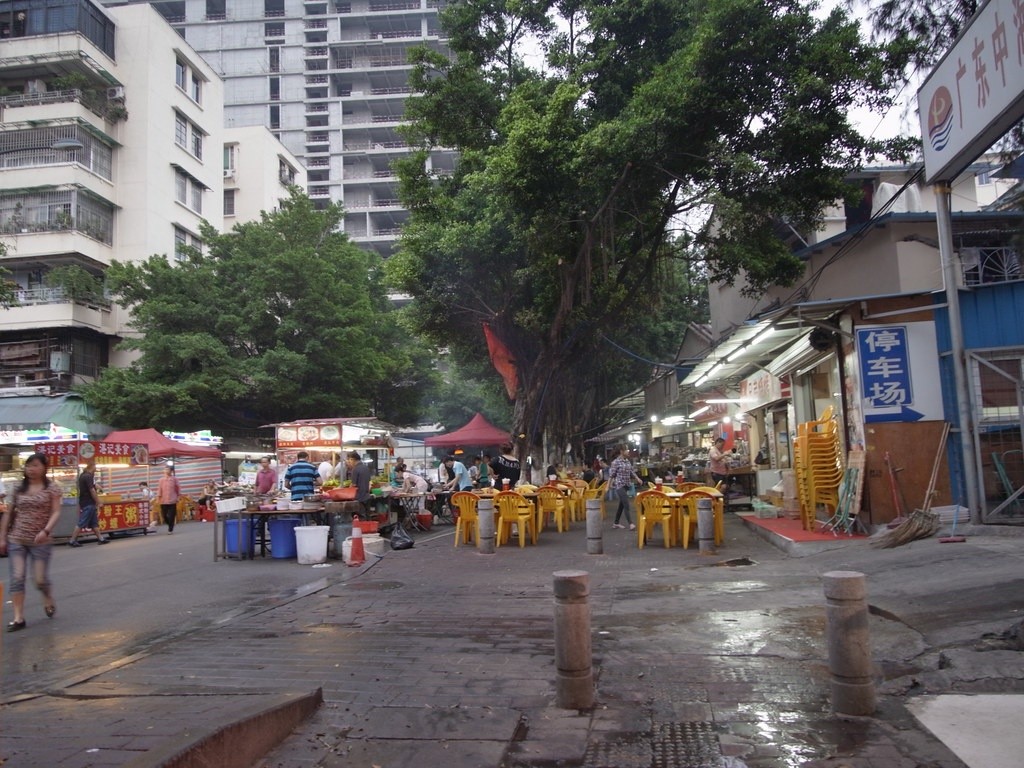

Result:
[98,539,110,545]
[254,536,261,543]
[411,521,419,528]
[68,540,83,547]
[44,605,56,617]
[7,619,26,631]
[629,523,636,530]
[168,531,172,534]
[613,523,625,529]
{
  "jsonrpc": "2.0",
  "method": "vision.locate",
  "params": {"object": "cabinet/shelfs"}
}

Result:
[720,472,755,511]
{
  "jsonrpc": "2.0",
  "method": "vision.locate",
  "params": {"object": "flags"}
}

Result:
[483,322,519,400]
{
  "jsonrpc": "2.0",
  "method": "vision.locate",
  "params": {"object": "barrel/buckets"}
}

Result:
[417,514,433,531]
[266,518,303,558]
[224,519,256,556]
[293,524,330,563]
[452,508,462,525]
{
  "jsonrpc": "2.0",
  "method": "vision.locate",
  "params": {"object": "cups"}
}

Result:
[655,484,662,492]
[503,484,509,492]
[677,475,683,483]
[548,480,555,487]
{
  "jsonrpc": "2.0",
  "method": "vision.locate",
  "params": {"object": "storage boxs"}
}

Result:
[389,512,398,524]
[225,518,257,554]
[764,469,801,519]
[215,496,247,513]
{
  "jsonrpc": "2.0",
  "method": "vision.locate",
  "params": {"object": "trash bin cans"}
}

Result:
[223,519,259,559]
[294,525,330,565]
[266,518,301,559]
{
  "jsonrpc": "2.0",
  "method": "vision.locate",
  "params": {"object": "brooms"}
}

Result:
[885,450,908,529]
[870,422,953,549]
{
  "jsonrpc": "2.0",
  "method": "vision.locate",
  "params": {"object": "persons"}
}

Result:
[475,455,492,488]
[156,465,181,535]
[582,459,595,489]
[439,453,473,518]
[198,479,218,510]
[334,454,352,480]
[487,441,521,534]
[732,439,741,454]
[391,457,407,505]
[139,482,156,510]
[709,437,731,505]
[595,454,611,494]
[547,460,568,480]
[68,461,111,547]
[394,466,429,529]
[284,452,323,501]
[605,443,643,530]
[0,453,62,632]
[349,451,371,521]
[253,456,278,536]
[318,454,340,481]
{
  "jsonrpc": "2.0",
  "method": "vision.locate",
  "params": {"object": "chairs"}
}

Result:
[451,475,608,547]
[633,481,725,551]
[149,493,214,524]
[793,403,847,534]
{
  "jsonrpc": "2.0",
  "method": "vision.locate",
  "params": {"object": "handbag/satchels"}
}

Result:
[0,495,17,557]
[390,523,415,549]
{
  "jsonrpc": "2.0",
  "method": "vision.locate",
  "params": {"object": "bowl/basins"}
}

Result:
[244,493,319,513]
[323,484,359,500]
[359,521,378,534]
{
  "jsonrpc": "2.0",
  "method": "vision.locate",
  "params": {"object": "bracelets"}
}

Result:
[43,528,49,536]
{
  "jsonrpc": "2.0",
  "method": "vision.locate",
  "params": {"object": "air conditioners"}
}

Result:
[222,170,232,178]
[106,86,125,101]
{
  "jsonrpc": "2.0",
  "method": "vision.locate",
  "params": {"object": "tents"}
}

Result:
[102,428,222,525]
[423,412,513,480]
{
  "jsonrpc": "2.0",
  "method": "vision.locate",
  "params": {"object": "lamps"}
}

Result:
[362,453,373,463]
[455,447,464,455]
[689,328,775,418]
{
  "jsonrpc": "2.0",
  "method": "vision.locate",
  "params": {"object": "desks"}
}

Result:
[469,492,541,544]
[637,491,723,546]
[663,483,679,486]
[545,487,569,528]
[570,484,587,522]
[819,449,872,537]
[393,491,455,533]
[213,508,325,562]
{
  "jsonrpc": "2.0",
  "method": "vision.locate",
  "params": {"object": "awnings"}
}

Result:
[679,300,854,388]
